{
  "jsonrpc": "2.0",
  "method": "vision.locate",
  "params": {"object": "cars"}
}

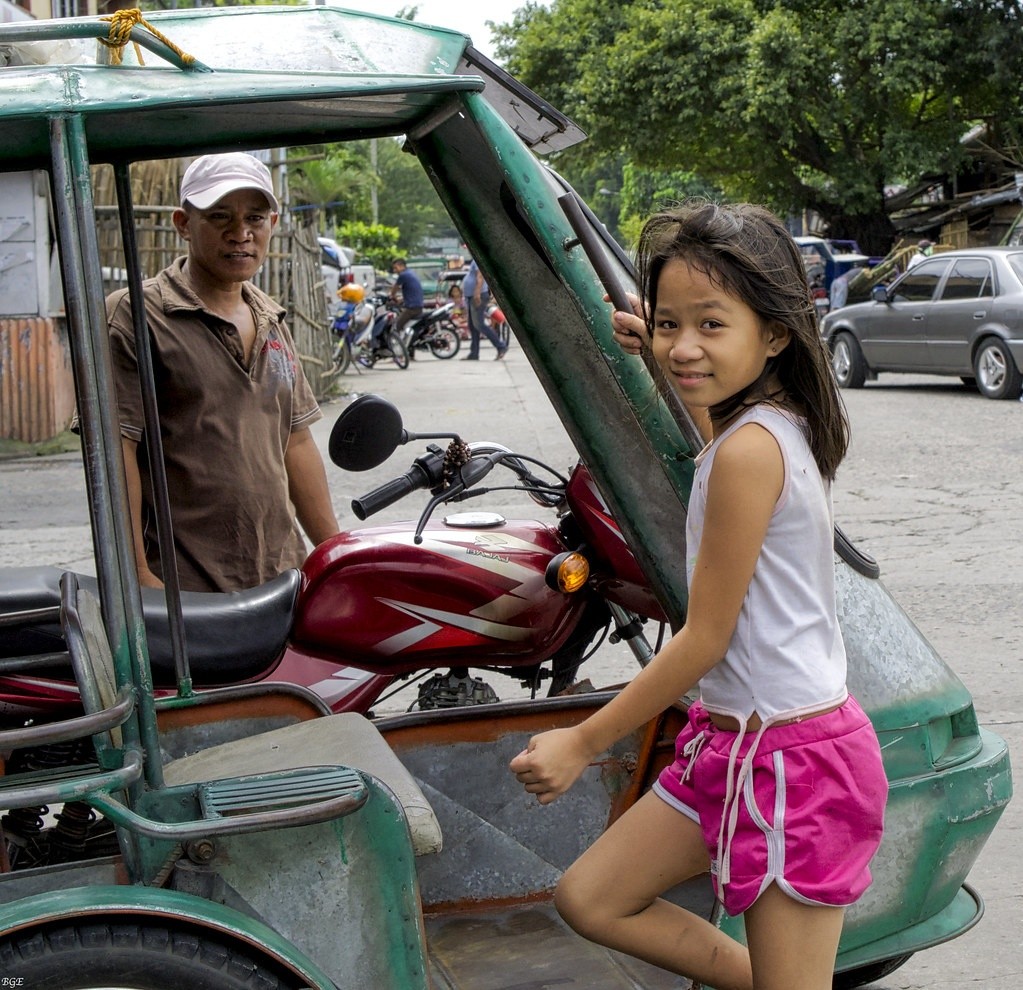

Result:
[817,244,1022,398]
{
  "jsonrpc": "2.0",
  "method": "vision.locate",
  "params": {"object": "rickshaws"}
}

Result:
[0,4,1014,990]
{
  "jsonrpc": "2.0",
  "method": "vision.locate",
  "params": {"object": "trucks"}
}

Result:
[404,257,451,308]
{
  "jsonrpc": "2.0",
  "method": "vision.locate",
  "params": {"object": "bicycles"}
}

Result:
[494,303,509,346]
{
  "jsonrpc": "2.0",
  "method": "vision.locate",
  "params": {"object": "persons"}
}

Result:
[71,151,340,596]
[462,259,508,360]
[512,204,889,990]
[907,240,936,270]
[391,258,423,331]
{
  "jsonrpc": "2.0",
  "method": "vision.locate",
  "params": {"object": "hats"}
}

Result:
[180,152,280,213]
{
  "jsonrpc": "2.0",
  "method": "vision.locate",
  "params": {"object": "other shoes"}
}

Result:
[460,356,478,361]
[495,348,508,361]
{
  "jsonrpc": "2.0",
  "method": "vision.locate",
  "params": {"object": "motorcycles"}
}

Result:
[369,283,460,360]
[330,282,409,377]
